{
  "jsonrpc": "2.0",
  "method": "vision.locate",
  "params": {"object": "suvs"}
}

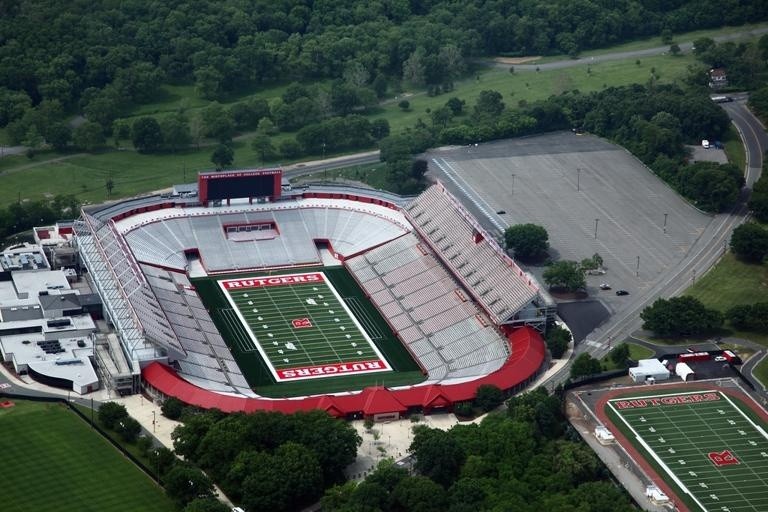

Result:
[715,356,726,363]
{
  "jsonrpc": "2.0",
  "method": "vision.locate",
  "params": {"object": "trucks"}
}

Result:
[711,96,732,104]
[713,140,723,149]
[701,139,710,149]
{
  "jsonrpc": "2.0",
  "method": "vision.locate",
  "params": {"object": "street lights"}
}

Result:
[511,174,517,196]
[722,238,728,255]
[634,255,641,277]
[593,219,600,239]
[576,167,581,192]
[690,268,697,287]
[663,212,668,235]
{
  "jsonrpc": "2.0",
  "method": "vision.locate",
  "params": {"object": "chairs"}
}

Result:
[83,191,536,401]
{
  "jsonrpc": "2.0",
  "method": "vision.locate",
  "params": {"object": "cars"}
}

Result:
[600,282,612,290]
[496,207,508,215]
[584,268,607,275]
[616,289,629,295]
